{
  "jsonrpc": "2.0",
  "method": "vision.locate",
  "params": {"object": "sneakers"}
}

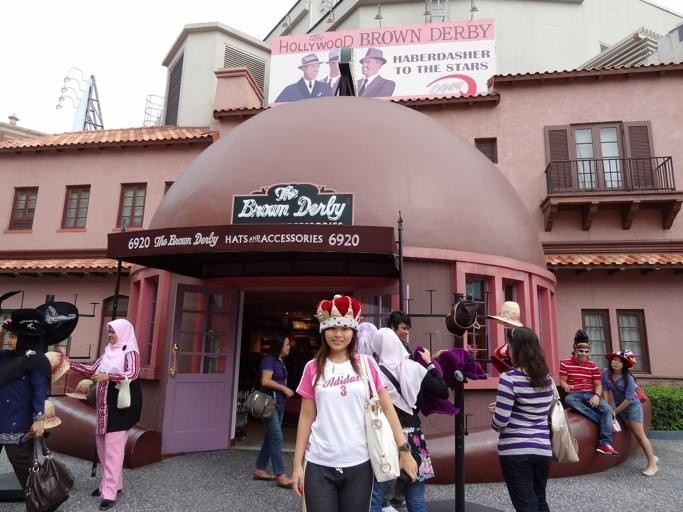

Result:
[596,444,618,456]
[643,455,659,476]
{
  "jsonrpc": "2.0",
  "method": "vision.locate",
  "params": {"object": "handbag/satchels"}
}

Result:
[114,375,132,409]
[636,387,647,402]
[550,401,580,464]
[365,397,400,483]
[242,390,275,420]
[23,435,74,512]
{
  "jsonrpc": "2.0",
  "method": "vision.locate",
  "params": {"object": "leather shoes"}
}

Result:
[99,499,115,510]
[276,479,292,488]
[254,472,274,480]
[91,488,122,496]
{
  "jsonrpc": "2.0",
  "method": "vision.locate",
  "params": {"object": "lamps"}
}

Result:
[279,0,480,35]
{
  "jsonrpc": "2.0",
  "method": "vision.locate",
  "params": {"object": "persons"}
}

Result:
[0,319,52,512]
[318,49,339,96]
[387,311,413,359]
[370,327,449,512]
[357,48,395,97]
[70,319,142,511]
[602,351,659,476]
[274,54,332,103]
[290,294,418,512]
[355,321,378,356]
[559,329,621,456]
[487,326,580,512]
[254,335,295,488]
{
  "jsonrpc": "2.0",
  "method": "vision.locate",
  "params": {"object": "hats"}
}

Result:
[44,346,70,384]
[446,298,480,339]
[358,48,387,64]
[64,379,94,400]
[574,329,590,348]
[325,49,340,63]
[35,301,79,346]
[298,53,323,70]
[2,308,47,336]
[313,294,362,333]
[488,301,523,328]
[605,349,636,368]
[43,400,62,429]
[491,344,513,373]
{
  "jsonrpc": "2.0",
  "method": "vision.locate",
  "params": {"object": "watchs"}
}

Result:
[399,442,411,451]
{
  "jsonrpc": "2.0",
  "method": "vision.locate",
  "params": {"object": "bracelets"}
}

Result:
[595,394,601,398]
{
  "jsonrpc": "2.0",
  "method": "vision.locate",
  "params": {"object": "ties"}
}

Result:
[308,81,312,88]
[358,79,368,95]
[328,79,332,85]
